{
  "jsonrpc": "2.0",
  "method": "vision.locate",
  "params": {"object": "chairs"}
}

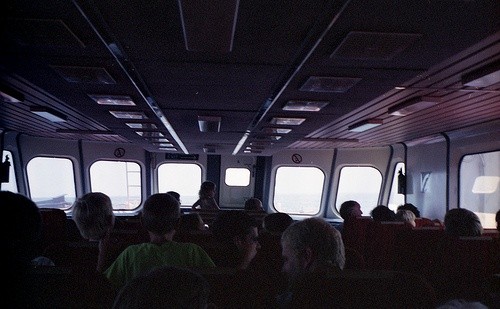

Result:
[41,207,500,309]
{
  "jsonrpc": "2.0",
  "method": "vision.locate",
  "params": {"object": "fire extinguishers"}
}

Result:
[398,168,405,194]
[1,155,11,183]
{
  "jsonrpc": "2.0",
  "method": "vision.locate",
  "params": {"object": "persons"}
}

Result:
[0,191,500,309]
[192,182,221,210]
